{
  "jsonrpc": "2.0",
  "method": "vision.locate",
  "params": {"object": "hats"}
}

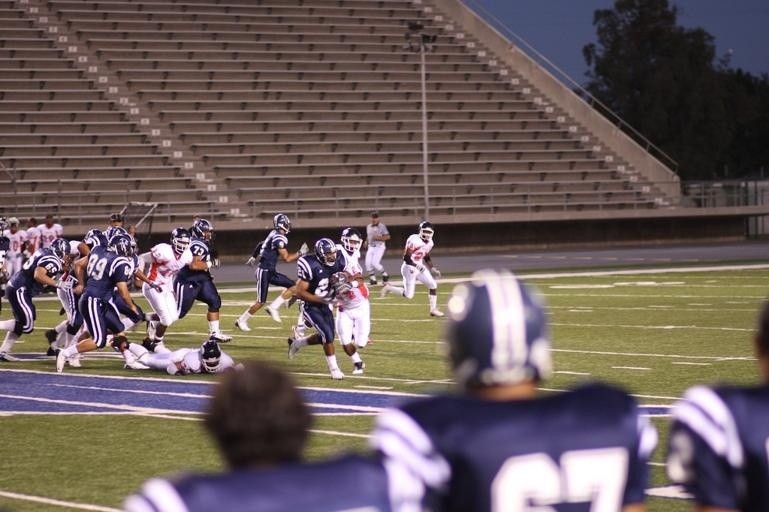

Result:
[110,213,122,221]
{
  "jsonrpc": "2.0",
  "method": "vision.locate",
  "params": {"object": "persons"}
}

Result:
[37,214,63,249]
[67,233,163,368]
[287,237,345,380]
[362,213,391,287]
[291,227,363,339]
[102,213,138,248]
[379,220,445,317]
[235,213,309,332]
[2,217,33,281]
[120,357,441,510]
[23,218,42,263]
[55,226,129,359]
[365,264,660,512]
[45,228,102,357]
[138,227,195,353]
[0,238,72,362]
[120,337,235,375]
[54,237,139,373]
[173,218,232,343]
[665,294,769,511]
[334,264,371,376]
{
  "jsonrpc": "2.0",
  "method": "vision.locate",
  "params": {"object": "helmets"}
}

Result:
[192,219,214,242]
[314,238,337,267]
[418,221,434,241]
[49,237,70,265]
[106,234,131,256]
[169,227,190,255]
[83,229,102,243]
[448,265,552,388]
[0,216,9,237]
[340,227,363,253]
[9,217,19,227]
[273,213,291,235]
[201,339,222,374]
[106,227,127,241]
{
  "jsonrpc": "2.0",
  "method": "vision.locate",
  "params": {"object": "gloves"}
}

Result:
[55,280,74,293]
[176,368,188,377]
[206,259,221,269]
[296,242,309,257]
[416,263,425,272]
[245,257,256,268]
[148,280,165,293]
[430,267,440,277]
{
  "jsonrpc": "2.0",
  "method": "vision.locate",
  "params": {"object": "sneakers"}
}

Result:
[264,306,282,323]
[125,361,150,370]
[208,331,233,342]
[140,337,153,350]
[150,340,171,354]
[0,351,19,362]
[67,353,81,368]
[352,361,365,375]
[46,329,57,352]
[287,336,299,360]
[330,368,345,380]
[147,321,156,341]
[56,348,66,373]
[235,319,251,332]
[47,347,62,356]
[380,283,390,298]
[429,309,444,317]
[291,325,305,340]
